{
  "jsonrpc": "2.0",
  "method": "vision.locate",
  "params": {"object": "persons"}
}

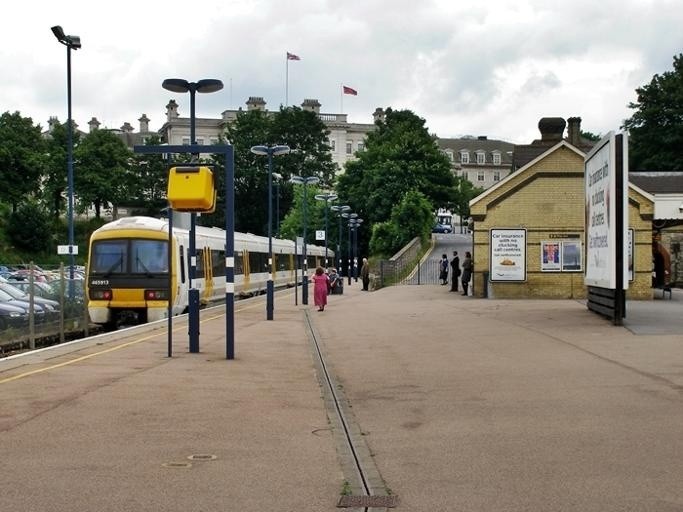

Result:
[324,269,329,295]
[450,251,458,292]
[360,258,369,292]
[328,268,339,293]
[460,252,471,296]
[439,254,448,285]
[309,266,329,311]
[543,244,558,263]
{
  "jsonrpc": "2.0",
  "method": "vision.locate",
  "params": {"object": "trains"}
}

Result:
[85,215,335,333]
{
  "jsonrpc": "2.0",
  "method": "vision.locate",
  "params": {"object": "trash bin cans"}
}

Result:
[332,278,343,294]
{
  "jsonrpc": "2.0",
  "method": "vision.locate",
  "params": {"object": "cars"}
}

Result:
[431,222,452,234]
[0,264,86,330]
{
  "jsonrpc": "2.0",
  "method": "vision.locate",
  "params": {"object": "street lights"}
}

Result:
[314,194,338,275]
[288,175,320,305]
[162,77,224,352]
[330,205,351,277]
[51,25,82,296]
[341,212,364,278]
[250,145,291,321]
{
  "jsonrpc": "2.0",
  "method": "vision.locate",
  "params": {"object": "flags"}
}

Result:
[343,86,357,96]
[287,53,299,61]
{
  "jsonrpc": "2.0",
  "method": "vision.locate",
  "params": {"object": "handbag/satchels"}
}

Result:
[457,268,461,276]
